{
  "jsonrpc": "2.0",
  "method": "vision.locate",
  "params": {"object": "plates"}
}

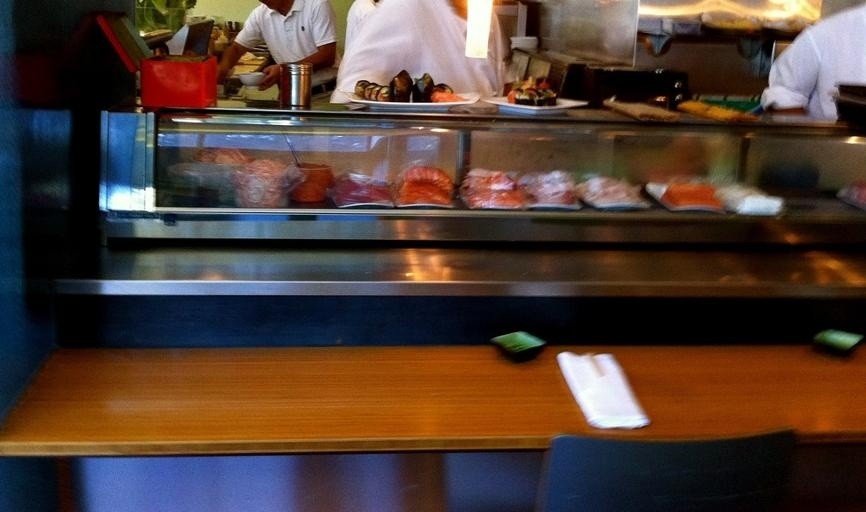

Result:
[328,188,396,209]
[395,198,457,209]
[460,193,522,211]
[646,182,727,215]
[510,36,539,52]
[583,196,653,210]
[344,90,481,112]
[481,96,590,118]
[523,199,583,212]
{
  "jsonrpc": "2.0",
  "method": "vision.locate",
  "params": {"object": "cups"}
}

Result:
[279,61,314,108]
[287,163,333,204]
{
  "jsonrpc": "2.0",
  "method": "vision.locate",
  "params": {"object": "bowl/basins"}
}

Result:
[238,72,267,90]
[489,330,548,364]
[811,327,865,356]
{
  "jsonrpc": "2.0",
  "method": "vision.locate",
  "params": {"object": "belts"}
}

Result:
[310,78,335,95]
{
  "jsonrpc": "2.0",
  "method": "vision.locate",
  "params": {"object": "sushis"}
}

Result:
[508,77,565,106]
[353,66,466,102]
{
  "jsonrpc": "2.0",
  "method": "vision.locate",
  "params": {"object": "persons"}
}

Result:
[759,2,866,120]
[329,0,516,102]
[217,0,341,91]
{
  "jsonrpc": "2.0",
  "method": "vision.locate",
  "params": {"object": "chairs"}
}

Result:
[546,426,800,512]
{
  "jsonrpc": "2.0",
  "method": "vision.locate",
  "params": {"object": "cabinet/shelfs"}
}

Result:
[103,91,866,251]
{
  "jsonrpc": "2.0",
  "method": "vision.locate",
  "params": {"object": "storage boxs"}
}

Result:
[96,11,217,108]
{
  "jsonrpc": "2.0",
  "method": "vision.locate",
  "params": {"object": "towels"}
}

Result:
[557,351,651,429]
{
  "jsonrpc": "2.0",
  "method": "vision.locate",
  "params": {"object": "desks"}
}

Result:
[2,346,866,456]
